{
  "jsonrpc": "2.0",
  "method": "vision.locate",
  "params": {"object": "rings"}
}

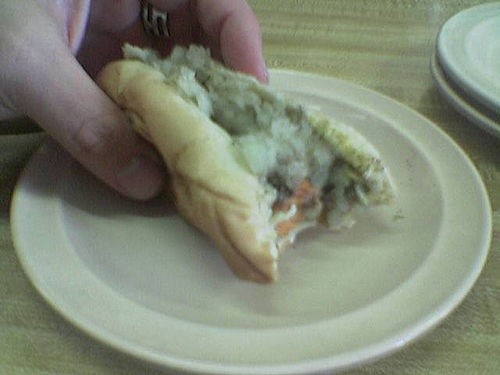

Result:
[141,3,171,42]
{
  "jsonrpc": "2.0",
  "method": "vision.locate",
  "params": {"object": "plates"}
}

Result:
[429,52,498,135]
[436,0,499,113]
[7,68,493,375]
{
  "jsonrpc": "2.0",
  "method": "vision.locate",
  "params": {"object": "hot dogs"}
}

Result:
[93,40,397,284]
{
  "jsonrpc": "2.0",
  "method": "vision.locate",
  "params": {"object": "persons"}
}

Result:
[0,1,270,203]
